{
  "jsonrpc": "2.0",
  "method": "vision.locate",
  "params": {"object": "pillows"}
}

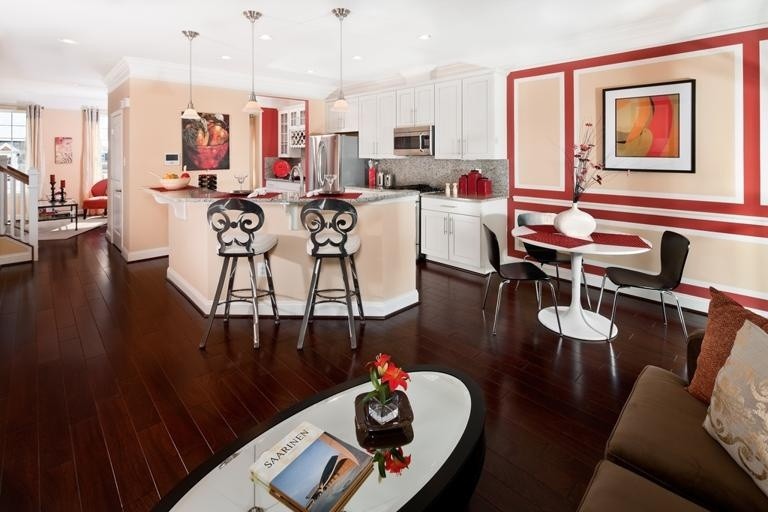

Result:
[700,321,767,493]
[688,286,768,398]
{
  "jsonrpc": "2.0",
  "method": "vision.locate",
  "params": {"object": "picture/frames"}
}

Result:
[181,111,230,171]
[55,137,73,164]
[603,80,697,174]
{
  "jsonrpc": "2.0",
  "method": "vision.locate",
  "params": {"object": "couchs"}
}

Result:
[83,179,108,219]
[573,331,768,511]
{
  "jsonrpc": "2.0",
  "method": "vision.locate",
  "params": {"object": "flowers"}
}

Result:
[358,354,411,412]
[569,117,634,203]
[368,448,412,482]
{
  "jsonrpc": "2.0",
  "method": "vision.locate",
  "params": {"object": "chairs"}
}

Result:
[297,199,365,351]
[199,198,280,348]
[480,223,564,338]
[596,231,691,342]
[519,212,595,313]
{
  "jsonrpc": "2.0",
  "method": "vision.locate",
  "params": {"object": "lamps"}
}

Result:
[180,31,200,121]
[332,8,351,113]
[243,11,263,116]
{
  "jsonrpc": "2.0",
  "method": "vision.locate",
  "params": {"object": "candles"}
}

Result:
[50,173,55,184]
[60,178,65,186]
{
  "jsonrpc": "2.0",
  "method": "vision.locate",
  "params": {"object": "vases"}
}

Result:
[355,391,414,449]
[553,203,597,238]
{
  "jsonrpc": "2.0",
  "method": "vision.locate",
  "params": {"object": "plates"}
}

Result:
[272,160,290,178]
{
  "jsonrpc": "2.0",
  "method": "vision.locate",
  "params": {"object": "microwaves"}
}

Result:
[393,125,435,156]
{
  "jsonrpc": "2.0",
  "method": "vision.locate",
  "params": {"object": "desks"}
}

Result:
[511,223,652,340]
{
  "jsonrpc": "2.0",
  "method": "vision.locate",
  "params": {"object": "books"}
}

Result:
[249,420,375,512]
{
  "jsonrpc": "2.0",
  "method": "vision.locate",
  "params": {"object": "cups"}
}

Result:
[324,174,338,194]
[233,175,248,194]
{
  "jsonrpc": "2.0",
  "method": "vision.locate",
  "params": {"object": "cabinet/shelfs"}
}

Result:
[421,192,508,274]
[397,82,435,127]
[325,95,358,132]
[277,106,290,159]
[290,105,306,147]
[434,70,507,159]
[359,88,407,159]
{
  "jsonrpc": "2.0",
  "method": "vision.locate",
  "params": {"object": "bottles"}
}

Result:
[452,183,458,197]
[459,174,468,197]
[478,178,492,195]
[468,168,482,198]
[445,183,450,197]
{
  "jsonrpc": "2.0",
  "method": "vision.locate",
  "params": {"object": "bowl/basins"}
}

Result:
[158,177,192,191]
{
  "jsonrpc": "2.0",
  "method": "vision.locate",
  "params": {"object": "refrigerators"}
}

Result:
[308,132,366,196]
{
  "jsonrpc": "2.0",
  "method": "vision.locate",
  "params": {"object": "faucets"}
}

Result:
[288,165,306,198]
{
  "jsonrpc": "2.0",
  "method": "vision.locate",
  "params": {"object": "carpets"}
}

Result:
[38,215,107,240]
